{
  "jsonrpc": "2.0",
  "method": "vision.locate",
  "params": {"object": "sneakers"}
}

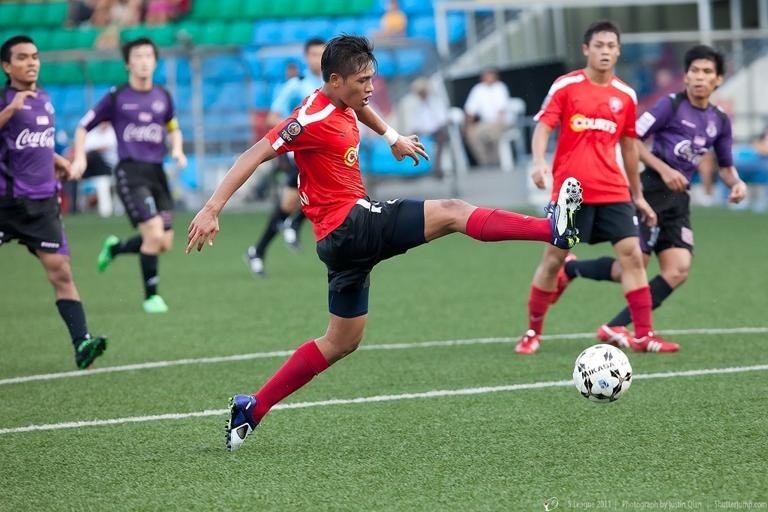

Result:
[597,323,631,351]
[97,234,120,271]
[549,252,577,304]
[549,177,583,251]
[226,394,254,452]
[283,218,298,246]
[72,334,105,370]
[246,245,264,275]
[515,327,541,356]
[141,293,168,314]
[631,330,680,352]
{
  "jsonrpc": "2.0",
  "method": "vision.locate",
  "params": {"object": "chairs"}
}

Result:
[2,2,526,217]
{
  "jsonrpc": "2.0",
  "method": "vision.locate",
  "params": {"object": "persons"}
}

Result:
[549,45,748,347]
[184,34,583,454]
[70,43,187,313]
[0,36,106,370]
[692,133,767,208]
[359,65,516,177]
[66,2,193,50]
[55,120,119,181]
[282,65,304,84]
[514,22,680,356]
[242,39,326,275]
[371,2,406,42]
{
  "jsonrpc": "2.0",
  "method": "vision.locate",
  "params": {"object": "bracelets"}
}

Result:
[381,126,399,145]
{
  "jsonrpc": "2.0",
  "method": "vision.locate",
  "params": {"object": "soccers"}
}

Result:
[572,343,632,404]
